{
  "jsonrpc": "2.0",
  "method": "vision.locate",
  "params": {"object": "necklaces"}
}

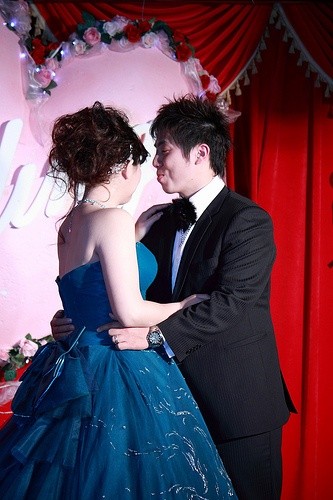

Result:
[77,199,107,209]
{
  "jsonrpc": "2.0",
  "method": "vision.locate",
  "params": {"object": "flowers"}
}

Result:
[7,0,222,109]
[0,334,53,381]
[167,198,196,233]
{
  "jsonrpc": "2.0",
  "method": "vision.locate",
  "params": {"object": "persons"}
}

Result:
[50,92,296,500]
[0,100,237,500]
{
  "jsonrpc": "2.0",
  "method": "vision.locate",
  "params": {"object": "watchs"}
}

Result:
[146,325,163,349]
